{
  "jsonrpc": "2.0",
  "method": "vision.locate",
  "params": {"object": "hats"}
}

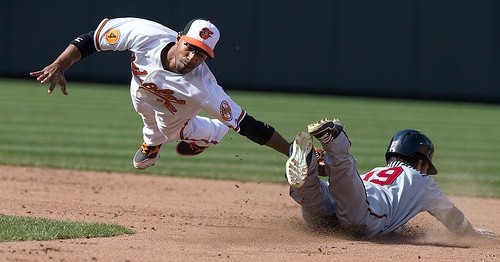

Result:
[180,19,220,59]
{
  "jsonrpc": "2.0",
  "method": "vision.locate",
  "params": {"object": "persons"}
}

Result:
[29,18,324,169]
[285,118,496,239]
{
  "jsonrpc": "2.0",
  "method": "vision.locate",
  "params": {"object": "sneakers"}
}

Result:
[132,141,162,170]
[176,140,209,156]
[285,132,313,188]
[307,117,352,149]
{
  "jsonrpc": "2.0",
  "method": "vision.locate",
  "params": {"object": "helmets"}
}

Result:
[385,129,438,175]
